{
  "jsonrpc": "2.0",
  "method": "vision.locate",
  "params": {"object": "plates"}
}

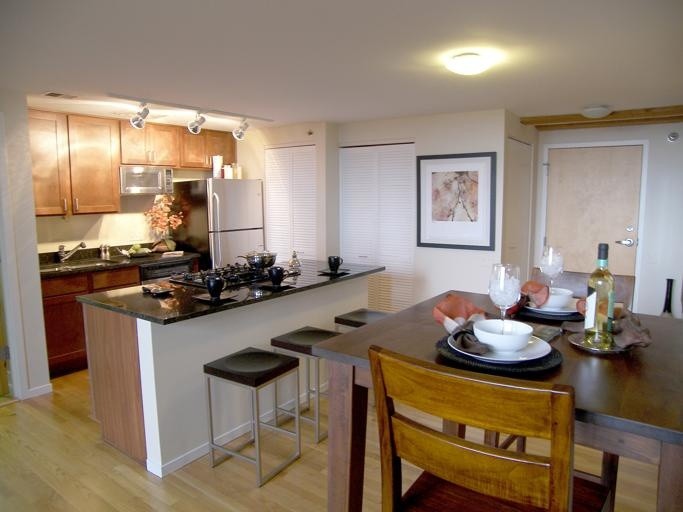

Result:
[433,335,563,374]
[256,284,295,293]
[523,297,588,316]
[191,292,238,304]
[160,250,185,259]
[118,248,153,257]
[567,331,634,358]
[317,267,349,277]
[445,328,553,365]
[515,312,582,324]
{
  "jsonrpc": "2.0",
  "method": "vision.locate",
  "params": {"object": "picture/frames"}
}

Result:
[415,149,498,250]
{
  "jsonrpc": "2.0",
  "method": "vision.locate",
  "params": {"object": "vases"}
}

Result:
[152,231,174,252]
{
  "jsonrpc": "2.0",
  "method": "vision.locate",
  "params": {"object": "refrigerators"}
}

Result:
[167,179,266,273]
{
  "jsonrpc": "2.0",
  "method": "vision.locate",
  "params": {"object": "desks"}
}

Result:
[311,285,680,512]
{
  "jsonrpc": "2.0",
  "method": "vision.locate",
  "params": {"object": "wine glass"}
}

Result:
[536,244,564,296]
[488,262,522,334]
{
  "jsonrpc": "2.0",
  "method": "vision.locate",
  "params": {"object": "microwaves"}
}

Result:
[118,164,173,195]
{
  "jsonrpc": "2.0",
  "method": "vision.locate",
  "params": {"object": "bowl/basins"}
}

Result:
[527,287,573,312]
[470,318,534,357]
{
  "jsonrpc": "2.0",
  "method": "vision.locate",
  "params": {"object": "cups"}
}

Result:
[599,301,624,323]
[266,266,287,286]
[205,276,227,296]
[529,268,551,290]
[327,255,342,271]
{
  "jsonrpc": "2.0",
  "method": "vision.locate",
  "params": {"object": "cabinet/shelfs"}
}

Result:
[43,269,139,373]
[179,126,237,170]
[118,120,179,169]
[26,106,121,216]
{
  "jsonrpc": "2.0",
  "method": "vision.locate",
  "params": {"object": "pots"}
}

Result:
[235,252,277,267]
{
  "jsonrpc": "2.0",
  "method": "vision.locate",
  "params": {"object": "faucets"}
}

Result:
[57,242,87,263]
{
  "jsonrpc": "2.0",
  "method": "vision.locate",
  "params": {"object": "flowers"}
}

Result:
[143,192,185,232]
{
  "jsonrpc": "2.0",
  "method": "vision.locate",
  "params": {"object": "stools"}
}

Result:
[204,342,302,489]
[332,309,390,331]
[270,321,342,444]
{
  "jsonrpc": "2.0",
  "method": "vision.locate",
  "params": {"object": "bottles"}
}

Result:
[583,242,615,350]
[100,244,110,262]
[211,152,242,179]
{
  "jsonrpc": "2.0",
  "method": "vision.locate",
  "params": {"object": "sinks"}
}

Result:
[39,264,70,273]
[66,261,122,269]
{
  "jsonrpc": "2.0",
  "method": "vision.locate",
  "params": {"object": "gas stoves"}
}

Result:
[169,263,300,289]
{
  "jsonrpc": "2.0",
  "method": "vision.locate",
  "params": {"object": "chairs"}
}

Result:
[364,343,613,512]
[530,267,637,312]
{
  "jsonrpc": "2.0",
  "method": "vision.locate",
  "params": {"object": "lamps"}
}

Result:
[128,104,252,142]
[583,103,612,121]
